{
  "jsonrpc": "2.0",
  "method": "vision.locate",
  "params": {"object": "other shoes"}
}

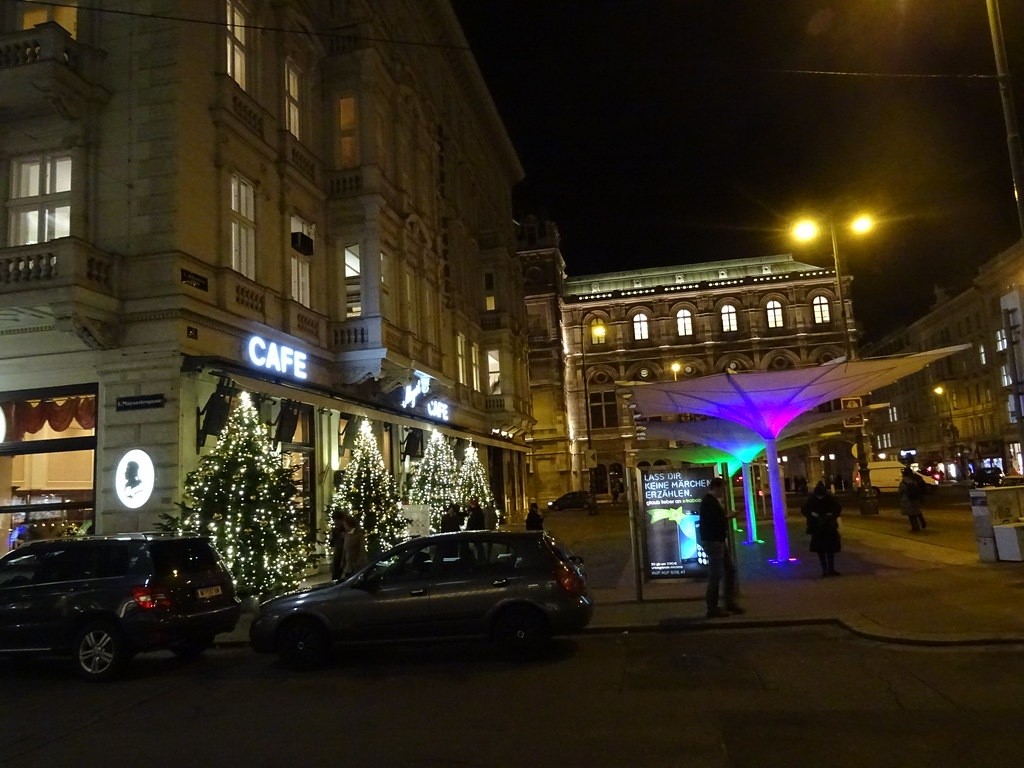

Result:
[707,607,747,618]
[820,570,840,576]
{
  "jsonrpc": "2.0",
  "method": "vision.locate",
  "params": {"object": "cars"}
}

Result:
[974,467,1002,485]
[248,529,594,671]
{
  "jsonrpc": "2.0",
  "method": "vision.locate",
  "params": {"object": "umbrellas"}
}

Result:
[616,343,971,567]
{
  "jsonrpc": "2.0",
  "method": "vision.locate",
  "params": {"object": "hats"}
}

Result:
[814,480,826,494]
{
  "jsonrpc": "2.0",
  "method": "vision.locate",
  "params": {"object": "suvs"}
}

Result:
[0,531,244,684]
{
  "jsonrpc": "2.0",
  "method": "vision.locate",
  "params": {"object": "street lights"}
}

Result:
[934,386,957,447]
[581,311,606,517]
[790,213,878,359]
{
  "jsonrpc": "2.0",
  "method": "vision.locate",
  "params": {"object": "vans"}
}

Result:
[852,461,938,498]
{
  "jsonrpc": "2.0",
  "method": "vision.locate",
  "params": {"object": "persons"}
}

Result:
[329,509,366,581]
[525,503,544,531]
[699,476,746,619]
[802,480,846,578]
[441,495,498,557]
